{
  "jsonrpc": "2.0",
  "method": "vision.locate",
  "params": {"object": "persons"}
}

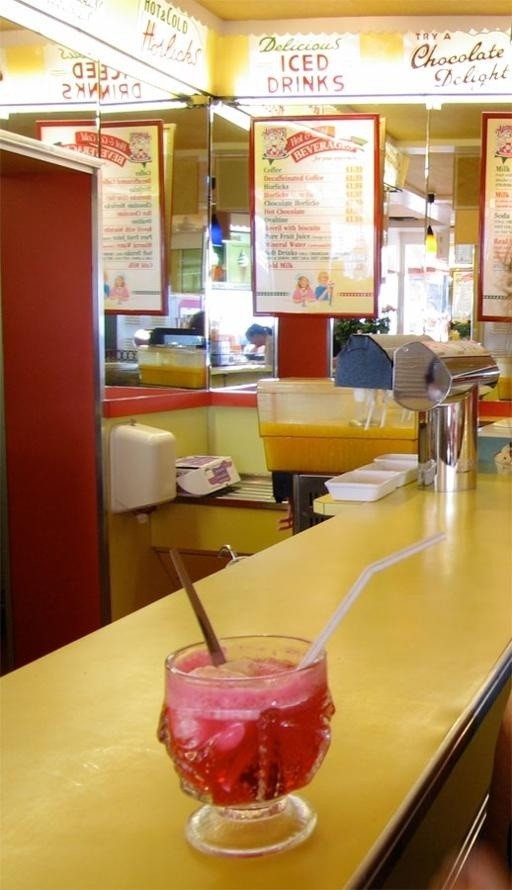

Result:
[189,310,221,366]
[245,322,274,364]
[104,271,129,302]
[292,271,334,306]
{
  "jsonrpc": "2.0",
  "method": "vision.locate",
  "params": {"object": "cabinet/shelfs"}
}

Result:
[147,499,292,591]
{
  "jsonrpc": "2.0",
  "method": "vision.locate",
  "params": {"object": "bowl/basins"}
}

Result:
[243,352,265,360]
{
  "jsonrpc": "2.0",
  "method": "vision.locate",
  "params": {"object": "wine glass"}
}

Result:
[156,636,335,856]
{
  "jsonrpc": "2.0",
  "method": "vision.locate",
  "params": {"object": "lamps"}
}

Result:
[211,214,222,246]
[424,107,438,257]
